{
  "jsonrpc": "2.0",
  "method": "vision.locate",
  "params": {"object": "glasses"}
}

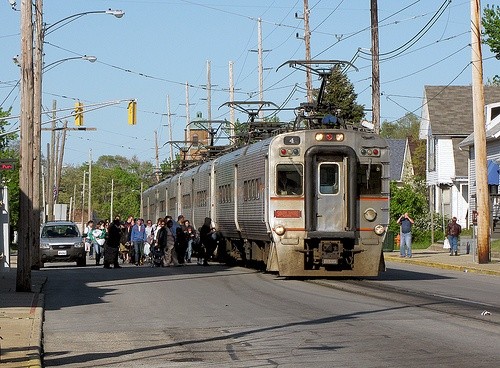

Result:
[162,223,165,225]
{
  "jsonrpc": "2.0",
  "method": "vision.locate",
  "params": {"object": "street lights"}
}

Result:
[32,9,126,272]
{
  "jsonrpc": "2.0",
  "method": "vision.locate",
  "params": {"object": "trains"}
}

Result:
[141,127,392,278]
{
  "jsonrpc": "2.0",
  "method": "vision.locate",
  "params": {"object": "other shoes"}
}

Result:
[455,250,458,255]
[197,262,203,265]
[114,265,121,268]
[450,253,454,256]
[203,263,210,266]
[103,266,112,269]
[187,259,192,263]
[135,262,139,266]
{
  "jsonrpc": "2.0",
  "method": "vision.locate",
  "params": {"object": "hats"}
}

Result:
[178,215,185,219]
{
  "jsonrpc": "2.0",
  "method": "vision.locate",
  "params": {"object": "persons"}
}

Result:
[396,213,415,258]
[83,215,218,269]
[278,172,299,191]
[445,217,462,256]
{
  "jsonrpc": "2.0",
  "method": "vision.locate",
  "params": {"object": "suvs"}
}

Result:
[39,219,88,267]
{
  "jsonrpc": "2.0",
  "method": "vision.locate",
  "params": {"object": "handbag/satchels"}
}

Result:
[143,243,151,255]
[443,236,451,249]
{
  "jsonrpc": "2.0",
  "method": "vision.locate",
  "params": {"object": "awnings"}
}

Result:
[473,160,500,186]
